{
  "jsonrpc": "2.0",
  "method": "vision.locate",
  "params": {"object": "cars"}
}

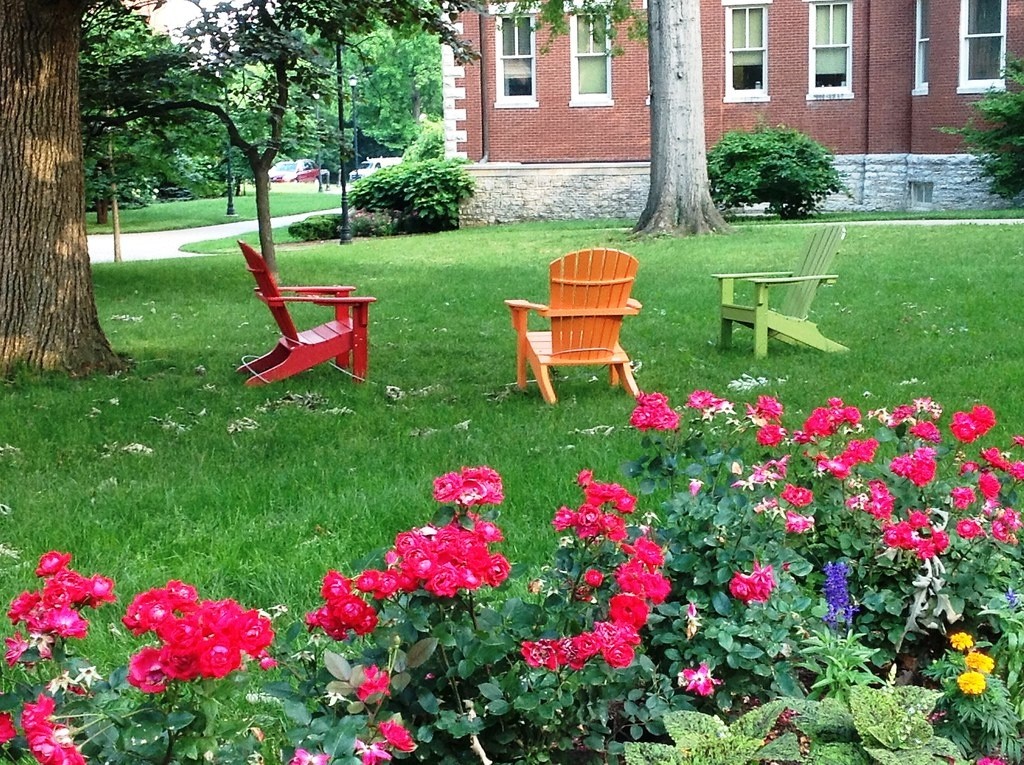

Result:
[267,159,321,186]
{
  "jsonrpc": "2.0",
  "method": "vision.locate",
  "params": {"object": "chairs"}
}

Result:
[504,248,644,406]
[711,224,851,358]
[236,239,377,386]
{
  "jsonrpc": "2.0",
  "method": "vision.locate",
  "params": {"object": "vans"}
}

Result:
[347,156,403,182]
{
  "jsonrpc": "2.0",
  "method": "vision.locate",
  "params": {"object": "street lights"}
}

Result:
[311,92,326,193]
[347,75,360,178]
